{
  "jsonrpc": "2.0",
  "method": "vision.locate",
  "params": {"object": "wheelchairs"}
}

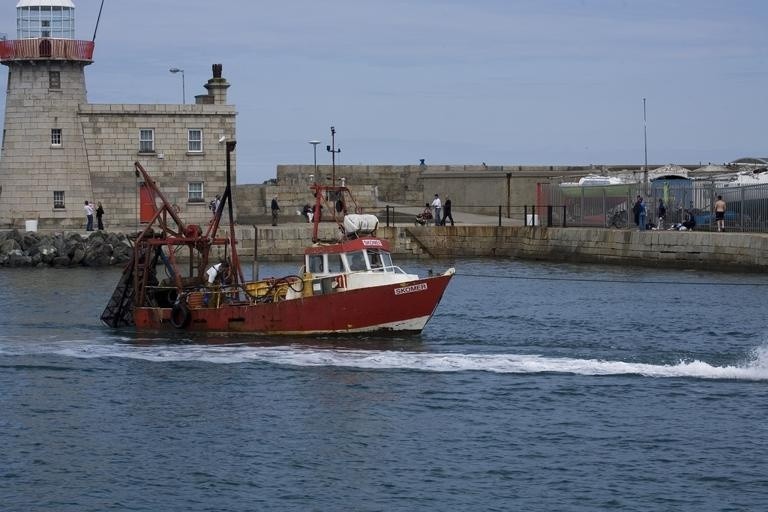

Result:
[414,206,435,226]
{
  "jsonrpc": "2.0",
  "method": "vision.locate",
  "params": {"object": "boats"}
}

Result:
[101,137,457,341]
[557,163,767,225]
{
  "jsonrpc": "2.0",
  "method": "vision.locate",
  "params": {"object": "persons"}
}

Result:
[84,200,94,231]
[210,194,219,223]
[422,194,454,226]
[312,204,323,222]
[203,261,230,308]
[714,195,727,232]
[633,194,696,231]
[95,201,105,230]
[270,195,281,226]
[215,195,222,228]
[302,201,314,223]
[350,254,365,269]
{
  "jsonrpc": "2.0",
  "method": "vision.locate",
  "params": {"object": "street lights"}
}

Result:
[326,126,342,219]
[169,68,185,104]
[308,140,321,182]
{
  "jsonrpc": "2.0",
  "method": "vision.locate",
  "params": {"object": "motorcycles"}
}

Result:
[605,208,628,229]
